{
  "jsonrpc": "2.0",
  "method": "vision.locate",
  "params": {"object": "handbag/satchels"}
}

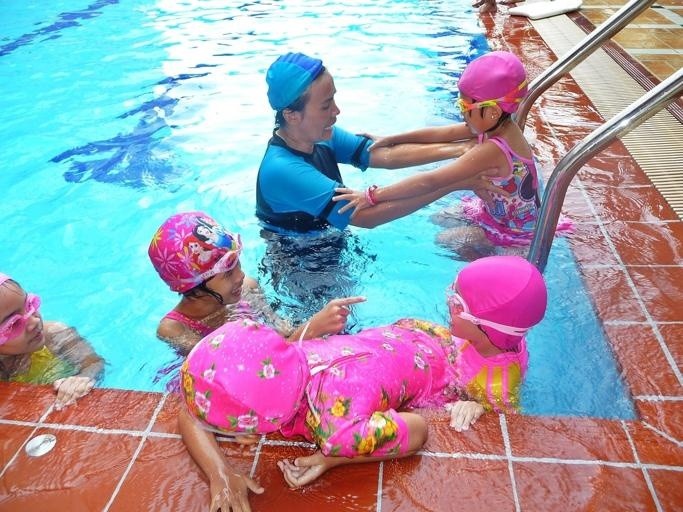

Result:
[365,181,383,209]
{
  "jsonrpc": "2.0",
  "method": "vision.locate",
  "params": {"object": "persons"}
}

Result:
[439,255,550,434]
[328,47,541,262]
[149,209,369,361]
[0,272,107,412]
[174,314,458,511]
[257,50,509,313]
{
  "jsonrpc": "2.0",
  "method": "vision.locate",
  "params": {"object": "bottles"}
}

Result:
[267,50,324,112]
[147,210,236,293]
[457,51,528,113]
[457,256,547,350]
[180,321,306,436]
[0,273,13,285]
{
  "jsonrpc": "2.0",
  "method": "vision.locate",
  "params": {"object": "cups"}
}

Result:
[0,293,42,344]
[445,283,479,325]
[457,99,497,112]
[179,232,243,283]
